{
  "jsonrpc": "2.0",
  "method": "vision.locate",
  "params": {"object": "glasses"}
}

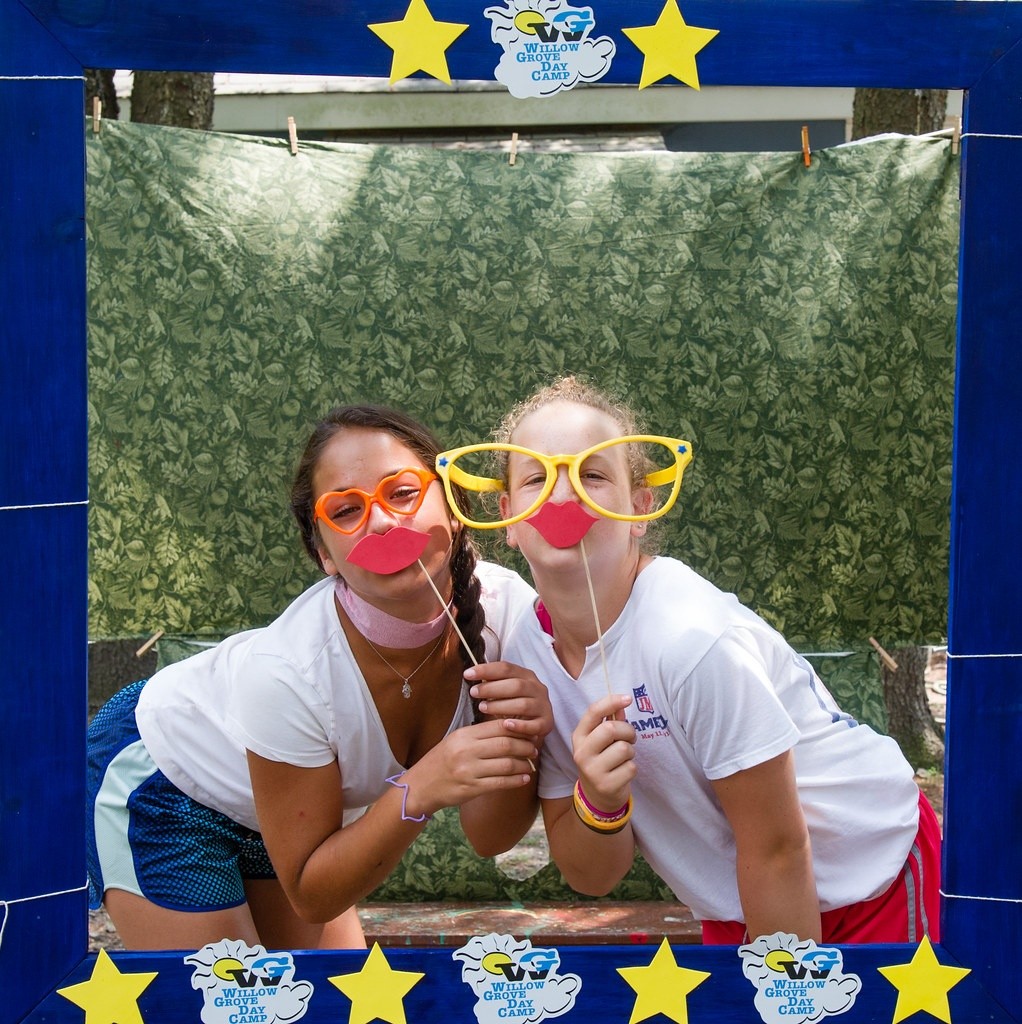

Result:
[314,468,439,536]
[436,436,693,529]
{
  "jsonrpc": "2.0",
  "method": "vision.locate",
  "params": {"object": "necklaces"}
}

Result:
[365,628,449,698]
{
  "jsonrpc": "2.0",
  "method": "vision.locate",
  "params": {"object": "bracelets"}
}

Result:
[572,777,633,835]
[385,769,432,822]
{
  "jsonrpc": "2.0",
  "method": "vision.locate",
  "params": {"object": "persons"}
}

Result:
[489,373,941,945]
[86,407,554,949]
